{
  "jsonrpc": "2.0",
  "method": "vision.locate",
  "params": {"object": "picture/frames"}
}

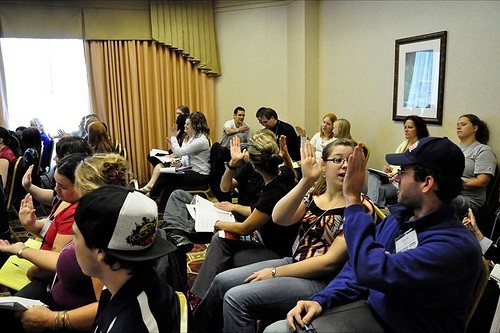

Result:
[392,30,447,125]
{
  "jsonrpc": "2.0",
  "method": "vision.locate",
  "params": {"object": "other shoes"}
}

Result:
[167,231,194,252]
[186,290,201,317]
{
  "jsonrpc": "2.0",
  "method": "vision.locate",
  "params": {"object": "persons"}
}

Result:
[463,207,500,333]
[189,138,376,333]
[0,106,302,333]
[366,116,429,207]
[265,136,483,333]
[295,114,337,163]
[321,119,357,148]
[451,113,496,223]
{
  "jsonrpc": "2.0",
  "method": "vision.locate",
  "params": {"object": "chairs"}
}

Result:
[6,133,54,242]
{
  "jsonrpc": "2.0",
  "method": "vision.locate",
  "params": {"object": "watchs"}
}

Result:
[272,267,276,278]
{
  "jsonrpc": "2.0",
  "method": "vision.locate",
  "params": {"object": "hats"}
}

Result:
[74,184,177,260]
[385,136,465,176]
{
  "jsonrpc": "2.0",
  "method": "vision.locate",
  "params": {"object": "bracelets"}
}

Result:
[18,246,30,259]
[52,310,73,332]
[224,162,237,170]
[214,220,220,232]
[237,129,239,133]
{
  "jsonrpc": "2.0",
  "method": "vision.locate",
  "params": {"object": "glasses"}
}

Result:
[325,157,348,163]
[398,168,416,174]
